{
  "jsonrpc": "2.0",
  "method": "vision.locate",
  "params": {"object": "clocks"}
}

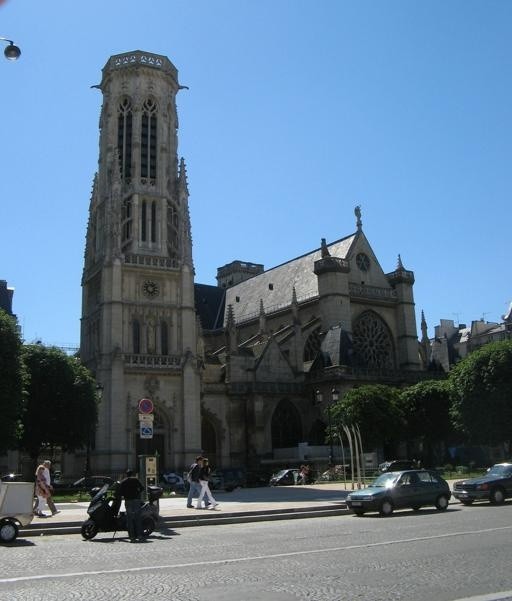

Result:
[142,280,159,298]
[357,253,370,270]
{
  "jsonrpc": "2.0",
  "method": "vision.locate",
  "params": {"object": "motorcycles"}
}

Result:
[80,483,163,539]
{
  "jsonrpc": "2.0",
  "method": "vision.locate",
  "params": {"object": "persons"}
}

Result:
[118,469,150,544]
[196,458,220,512]
[296,463,316,486]
[34,464,49,519]
[185,456,212,509]
[32,460,62,517]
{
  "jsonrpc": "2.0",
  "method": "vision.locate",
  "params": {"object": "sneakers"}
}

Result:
[187,502,218,510]
[33,511,61,517]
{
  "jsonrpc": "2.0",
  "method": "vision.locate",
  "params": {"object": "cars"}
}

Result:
[378,459,415,473]
[452,462,512,505]
[345,470,452,517]
[72,476,112,487]
[0,481,39,543]
[268,469,303,486]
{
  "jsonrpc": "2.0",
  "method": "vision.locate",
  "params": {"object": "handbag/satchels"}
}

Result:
[187,474,193,483]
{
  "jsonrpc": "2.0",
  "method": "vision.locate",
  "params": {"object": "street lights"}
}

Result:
[315,385,339,481]
